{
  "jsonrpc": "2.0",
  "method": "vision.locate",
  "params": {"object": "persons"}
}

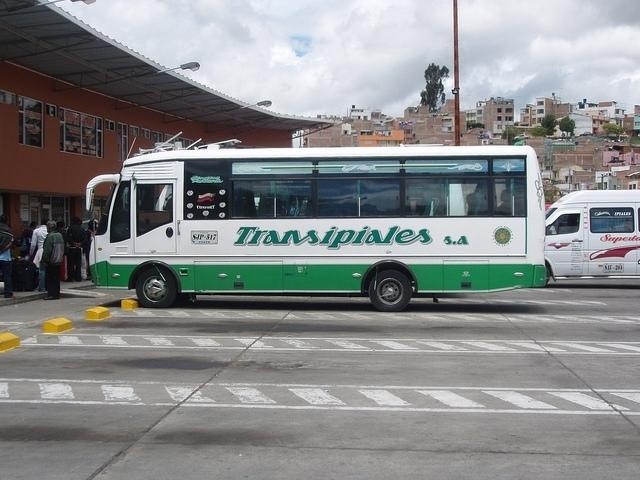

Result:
[497,188,509,215]
[0,215,15,299]
[38,219,65,300]
[289,190,307,216]
[0,214,100,292]
[465,193,476,216]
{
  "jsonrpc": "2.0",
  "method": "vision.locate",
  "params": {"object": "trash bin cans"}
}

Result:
[580,182,588,189]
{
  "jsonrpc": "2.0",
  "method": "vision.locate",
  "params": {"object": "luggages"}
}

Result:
[59,256,68,280]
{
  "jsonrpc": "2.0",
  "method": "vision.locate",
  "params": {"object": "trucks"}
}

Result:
[544,186,640,286]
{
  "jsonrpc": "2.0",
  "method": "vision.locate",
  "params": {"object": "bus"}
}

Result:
[81,131,549,313]
